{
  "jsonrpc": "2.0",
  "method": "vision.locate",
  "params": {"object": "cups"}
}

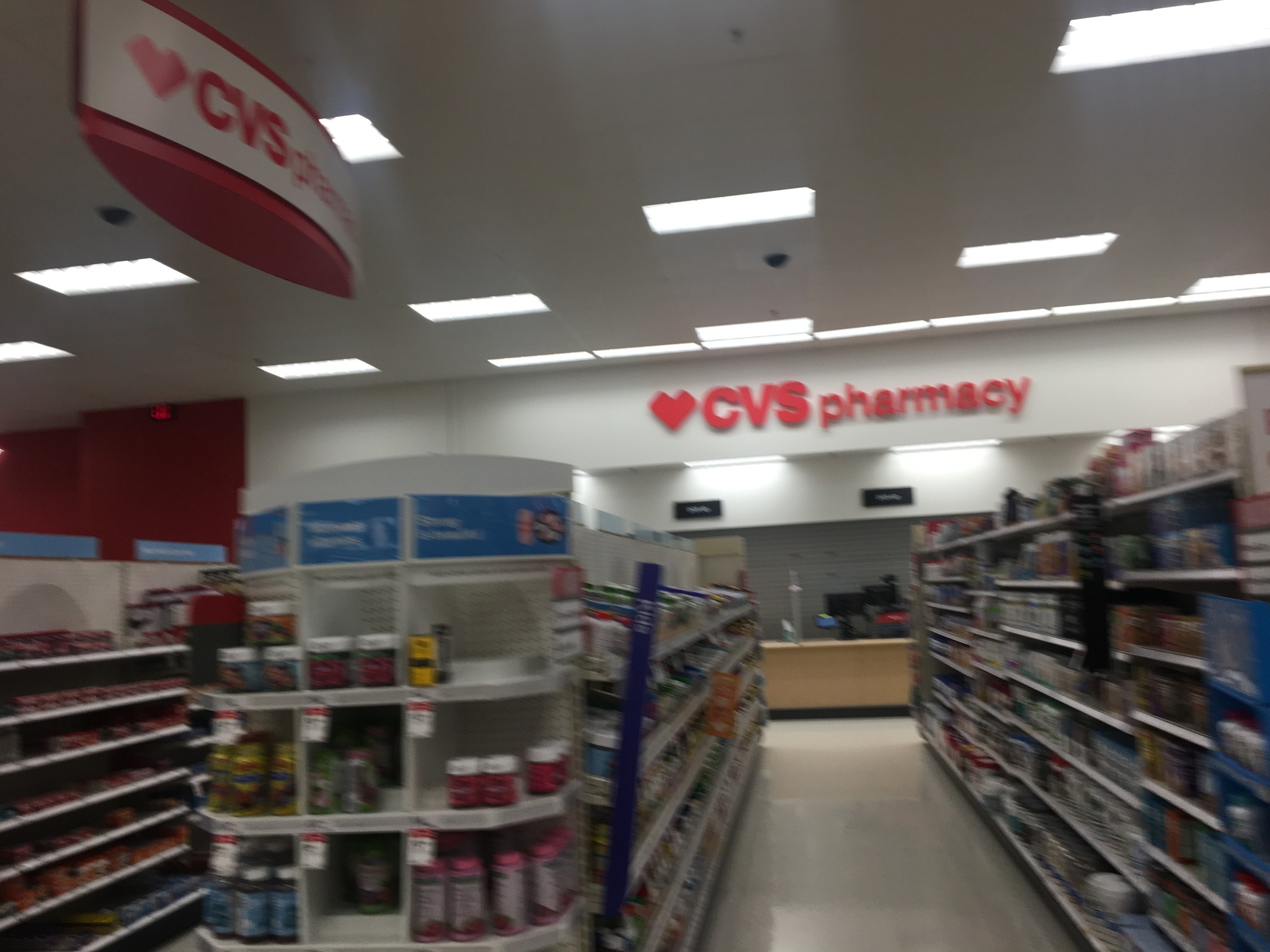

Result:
[582,726,620,778]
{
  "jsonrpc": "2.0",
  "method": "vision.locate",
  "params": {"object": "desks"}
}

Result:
[760,634,916,716]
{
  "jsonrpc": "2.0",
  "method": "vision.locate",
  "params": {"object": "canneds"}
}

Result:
[525,743,568,794]
[207,865,300,946]
[444,755,518,808]
[216,598,397,691]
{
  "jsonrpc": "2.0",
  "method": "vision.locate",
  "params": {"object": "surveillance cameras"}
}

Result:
[96,207,133,225]
[763,253,792,269]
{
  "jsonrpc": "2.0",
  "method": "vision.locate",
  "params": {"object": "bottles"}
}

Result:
[351,834,394,913]
[413,818,583,940]
[445,739,573,809]
[203,834,298,943]
[308,724,397,813]
[585,582,675,659]
[204,710,295,817]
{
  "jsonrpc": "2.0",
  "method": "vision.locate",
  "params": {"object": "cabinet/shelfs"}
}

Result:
[906,417,1270,952]
[0,556,765,952]
